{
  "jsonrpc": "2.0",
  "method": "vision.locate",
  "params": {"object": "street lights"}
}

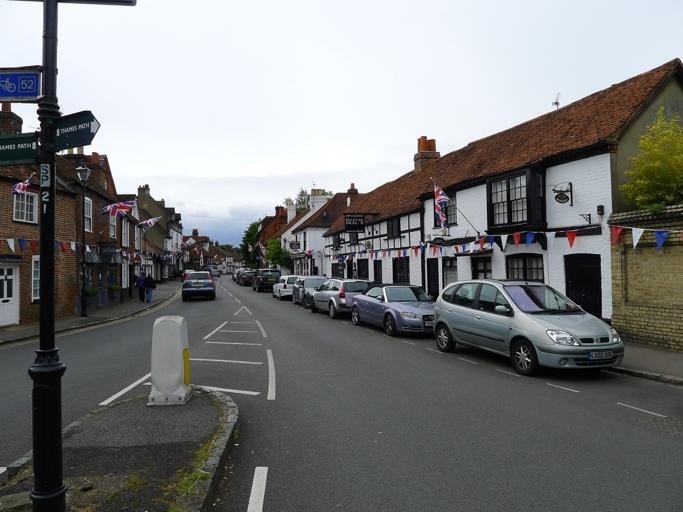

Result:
[75,158,90,319]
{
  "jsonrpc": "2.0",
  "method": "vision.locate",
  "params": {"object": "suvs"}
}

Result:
[250,268,281,291]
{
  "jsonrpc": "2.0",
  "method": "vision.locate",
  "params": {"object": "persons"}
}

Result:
[144,273,156,303]
[136,274,146,302]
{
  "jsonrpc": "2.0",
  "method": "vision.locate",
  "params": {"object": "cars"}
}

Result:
[308,276,371,318]
[231,267,255,286]
[348,283,437,338]
[181,272,215,300]
[180,269,194,280]
[209,269,220,277]
[290,276,328,308]
[270,275,299,300]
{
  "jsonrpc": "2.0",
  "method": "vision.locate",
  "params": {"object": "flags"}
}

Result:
[139,216,162,238]
[11,179,30,194]
[435,182,449,231]
[101,200,134,217]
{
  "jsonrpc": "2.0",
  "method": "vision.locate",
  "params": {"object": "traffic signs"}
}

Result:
[0,133,36,164]
[0,67,38,102]
[50,111,100,151]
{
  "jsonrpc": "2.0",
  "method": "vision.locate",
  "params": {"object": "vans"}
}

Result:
[430,279,625,380]
